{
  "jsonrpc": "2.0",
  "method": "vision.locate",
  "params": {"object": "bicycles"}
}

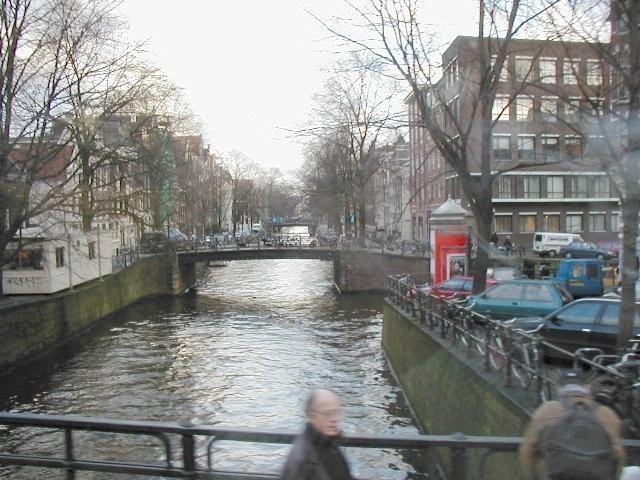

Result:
[215,233,426,253]
[394,271,544,397]
[560,334,639,421]
[500,245,526,259]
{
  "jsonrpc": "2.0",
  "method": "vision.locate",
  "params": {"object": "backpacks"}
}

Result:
[534,395,623,479]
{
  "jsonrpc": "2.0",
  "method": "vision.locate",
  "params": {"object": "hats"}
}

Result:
[556,369,582,385]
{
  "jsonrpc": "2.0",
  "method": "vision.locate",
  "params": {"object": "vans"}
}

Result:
[142,229,194,252]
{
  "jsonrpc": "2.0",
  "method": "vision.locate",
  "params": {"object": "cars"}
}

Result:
[407,260,640,363]
[532,232,619,259]
[202,223,261,243]
[288,215,298,223]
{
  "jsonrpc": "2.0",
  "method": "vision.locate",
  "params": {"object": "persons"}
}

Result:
[518,367,625,479]
[504,234,513,248]
[491,230,499,246]
[281,388,357,480]
